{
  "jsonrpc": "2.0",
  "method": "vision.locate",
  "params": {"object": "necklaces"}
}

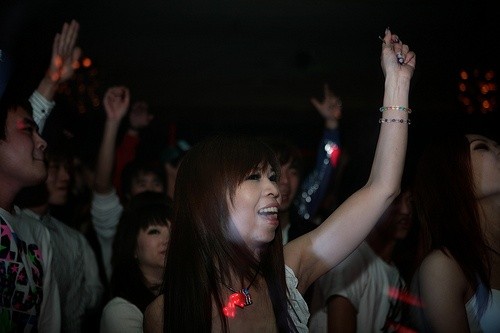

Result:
[481,241,500,256]
[220,261,263,319]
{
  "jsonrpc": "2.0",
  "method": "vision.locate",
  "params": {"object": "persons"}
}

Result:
[144,27,415,333]
[93,83,412,333]
[418,134,500,333]
[0,19,104,333]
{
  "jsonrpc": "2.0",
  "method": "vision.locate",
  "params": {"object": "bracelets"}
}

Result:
[379,105,412,114]
[378,118,411,125]
